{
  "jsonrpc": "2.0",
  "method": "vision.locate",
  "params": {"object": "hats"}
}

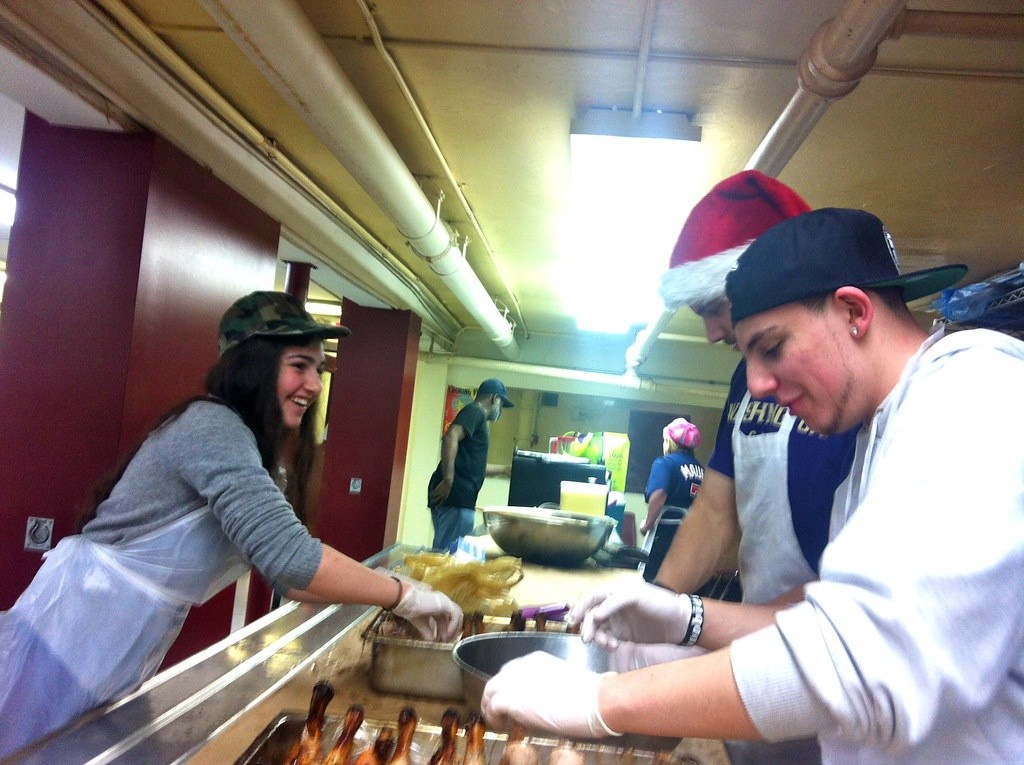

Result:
[725,206,968,331]
[668,417,700,448]
[477,378,515,408]
[214,291,352,356]
[657,169,814,308]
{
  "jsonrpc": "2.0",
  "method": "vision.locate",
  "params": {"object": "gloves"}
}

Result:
[569,572,694,650]
[392,583,464,643]
[479,650,625,738]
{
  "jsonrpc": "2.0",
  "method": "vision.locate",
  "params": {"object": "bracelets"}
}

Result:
[680,592,705,647]
[383,575,403,612]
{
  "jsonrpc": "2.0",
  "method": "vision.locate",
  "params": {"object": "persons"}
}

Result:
[0,290,463,765]
[426,378,512,555]
[638,417,707,584]
[652,169,865,764]
[480,207,1023,764]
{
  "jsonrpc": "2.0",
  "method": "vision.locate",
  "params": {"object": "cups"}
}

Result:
[560,480,608,516]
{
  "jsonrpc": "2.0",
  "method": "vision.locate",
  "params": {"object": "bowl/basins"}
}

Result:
[483,506,618,568]
[450,630,687,748]
